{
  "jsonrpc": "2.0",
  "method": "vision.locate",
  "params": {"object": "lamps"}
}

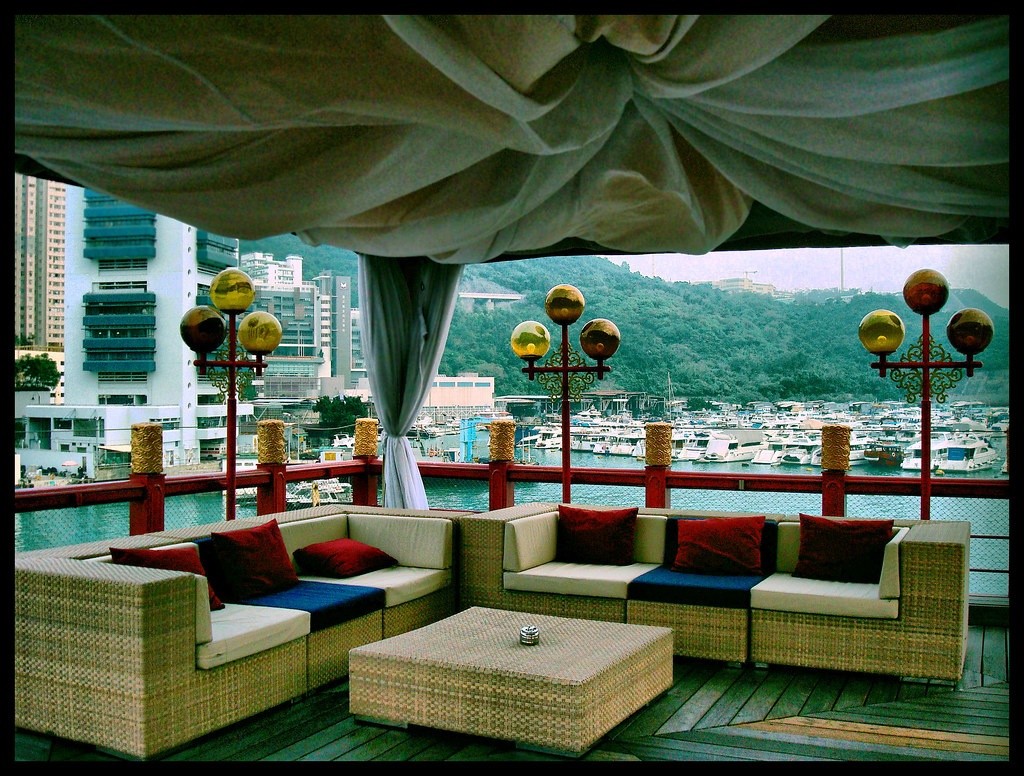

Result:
[857,269,994,521]
[511,283,622,505]
[178,267,283,522]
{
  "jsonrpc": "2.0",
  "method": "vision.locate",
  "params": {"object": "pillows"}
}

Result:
[555,503,639,565]
[210,518,300,598]
[790,509,895,586]
[293,538,399,579]
[106,546,226,611]
[673,514,769,576]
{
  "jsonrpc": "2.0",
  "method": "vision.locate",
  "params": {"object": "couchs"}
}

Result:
[463,500,973,686]
[14,505,474,763]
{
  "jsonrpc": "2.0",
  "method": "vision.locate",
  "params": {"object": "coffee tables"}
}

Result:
[347,606,675,759]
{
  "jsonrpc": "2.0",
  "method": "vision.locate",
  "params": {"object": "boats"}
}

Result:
[516,401,1010,467]
[287,482,351,504]
[899,435,950,473]
[935,429,1000,472]
